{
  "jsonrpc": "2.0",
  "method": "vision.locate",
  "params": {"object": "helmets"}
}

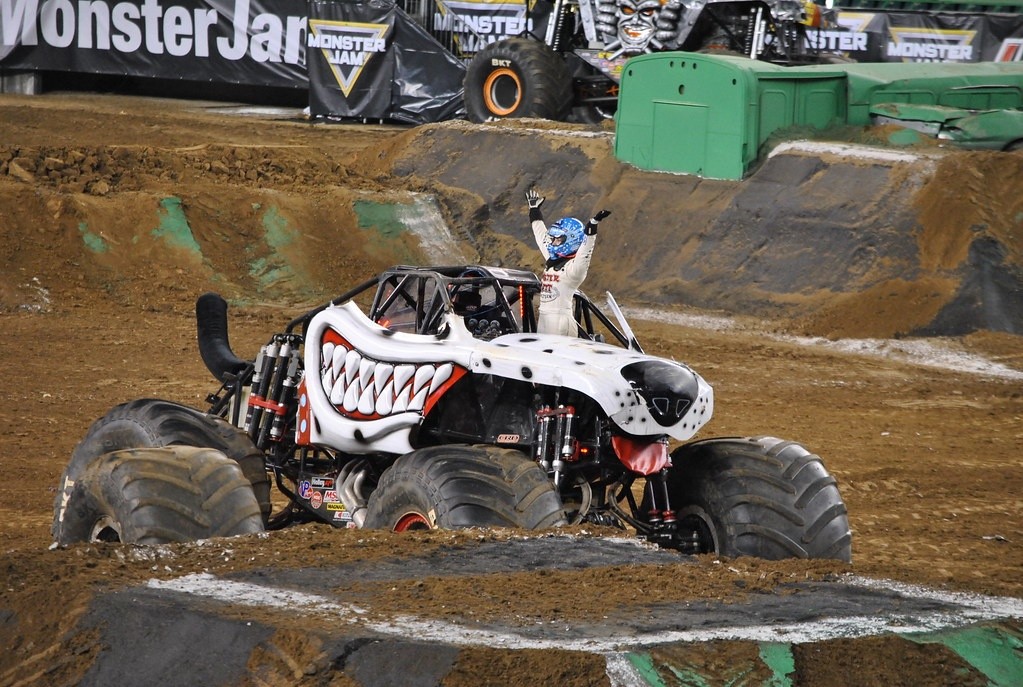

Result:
[543,217,586,260]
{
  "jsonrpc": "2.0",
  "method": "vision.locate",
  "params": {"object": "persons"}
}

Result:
[525,190,611,337]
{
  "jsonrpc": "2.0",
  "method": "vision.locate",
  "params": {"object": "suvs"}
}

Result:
[461,0,858,132]
[56,262,858,573]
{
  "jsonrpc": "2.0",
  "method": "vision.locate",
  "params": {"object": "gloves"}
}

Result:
[584,209,612,235]
[525,190,546,223]
